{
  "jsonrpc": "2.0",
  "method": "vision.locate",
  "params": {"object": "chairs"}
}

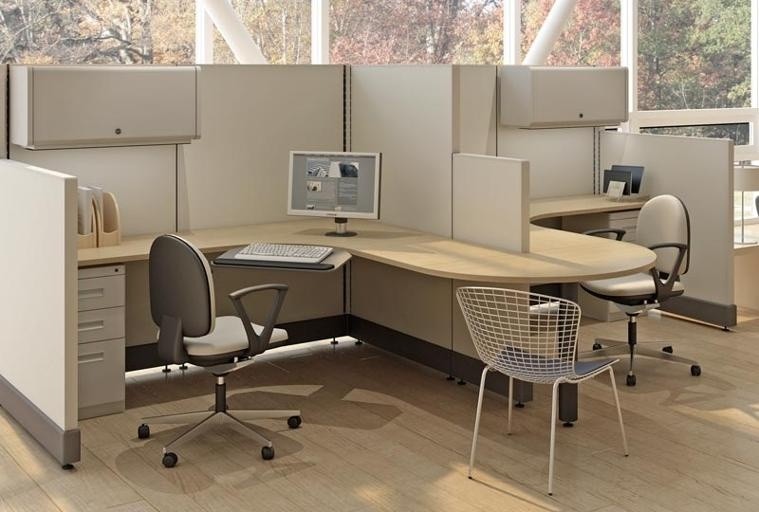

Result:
[579,194,701,386]
[455,287,630,491]
[135,234,303,468]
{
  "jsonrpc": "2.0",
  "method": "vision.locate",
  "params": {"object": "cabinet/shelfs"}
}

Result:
[78,263,127,420]
[562,210,640,322]
[8,63,200,151]
[501,64,628,127]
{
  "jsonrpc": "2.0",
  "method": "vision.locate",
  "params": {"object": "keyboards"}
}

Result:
[233,240,334,265]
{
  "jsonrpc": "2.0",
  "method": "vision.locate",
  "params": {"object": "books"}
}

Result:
[77,186,91,235]
[90,185,105,232]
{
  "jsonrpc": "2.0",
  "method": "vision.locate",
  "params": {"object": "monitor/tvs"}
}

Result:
[285,150,383,237]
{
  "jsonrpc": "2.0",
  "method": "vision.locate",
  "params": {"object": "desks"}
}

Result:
[528,194,651,324]
[79,216,595,419]
